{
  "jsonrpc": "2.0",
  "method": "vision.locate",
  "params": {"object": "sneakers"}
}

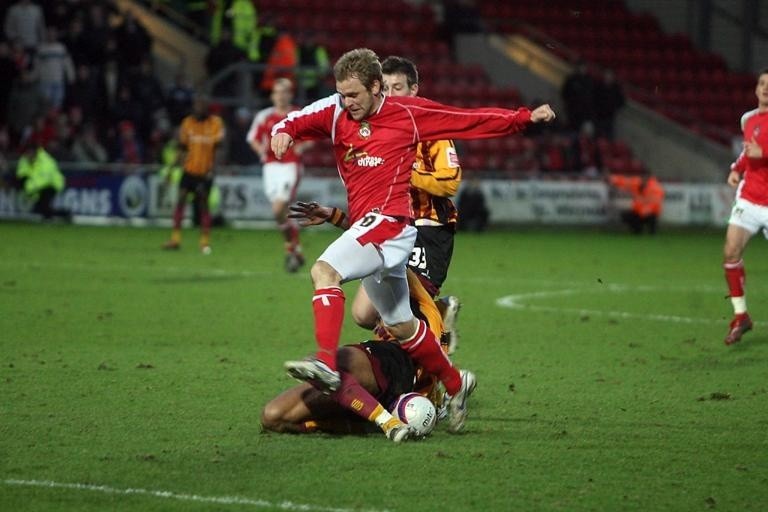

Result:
[725,316,753,345]
[385,421,434,443]
[284,357,343,397]
[435,368,477,433]
[439,295,464,356]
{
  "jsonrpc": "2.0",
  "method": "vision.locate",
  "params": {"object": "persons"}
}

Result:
[593,68,626,172]
[605,169,664,234]
[722,70,768,344]
[350,55,465,355]
[245,76,319,273]
[0,1,331,227]
[562,57,594,171]
[270,48,559,435]
[159,100,225,258]
[524,99,584,175]
[260,200,448,444]
[435,0,485,63]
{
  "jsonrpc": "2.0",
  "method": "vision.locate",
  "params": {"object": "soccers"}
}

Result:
[389,392,436,441]
